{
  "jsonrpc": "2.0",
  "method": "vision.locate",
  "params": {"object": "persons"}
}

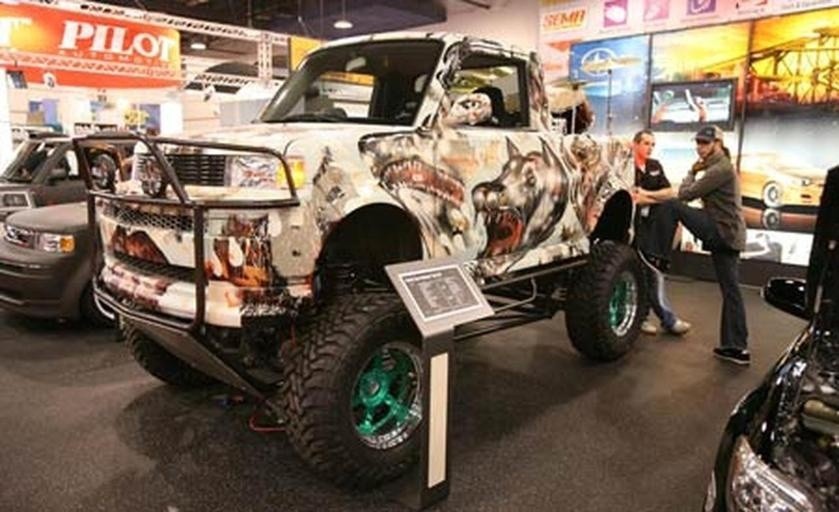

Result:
[638,126,750,366]
[633,130,691,335]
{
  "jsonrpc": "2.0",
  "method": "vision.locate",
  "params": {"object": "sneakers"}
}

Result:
[713,347,751,365]
[638,246,671,276]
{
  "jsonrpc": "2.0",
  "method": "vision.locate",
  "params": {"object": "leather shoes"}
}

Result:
[669,320,690,335]
[641,321,657,335]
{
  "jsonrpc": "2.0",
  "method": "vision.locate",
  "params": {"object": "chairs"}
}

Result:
[471,86,521,128]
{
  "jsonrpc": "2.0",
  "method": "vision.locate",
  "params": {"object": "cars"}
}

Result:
[700,166,839,512]
[0,132,144,331]
[733,146,825,209]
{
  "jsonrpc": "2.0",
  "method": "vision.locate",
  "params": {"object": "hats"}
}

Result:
[690,126,723,142]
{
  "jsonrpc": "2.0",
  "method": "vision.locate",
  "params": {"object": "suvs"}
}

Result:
[72,30,652,493]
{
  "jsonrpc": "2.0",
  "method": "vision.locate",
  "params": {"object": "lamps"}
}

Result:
[334,0,354,30]
